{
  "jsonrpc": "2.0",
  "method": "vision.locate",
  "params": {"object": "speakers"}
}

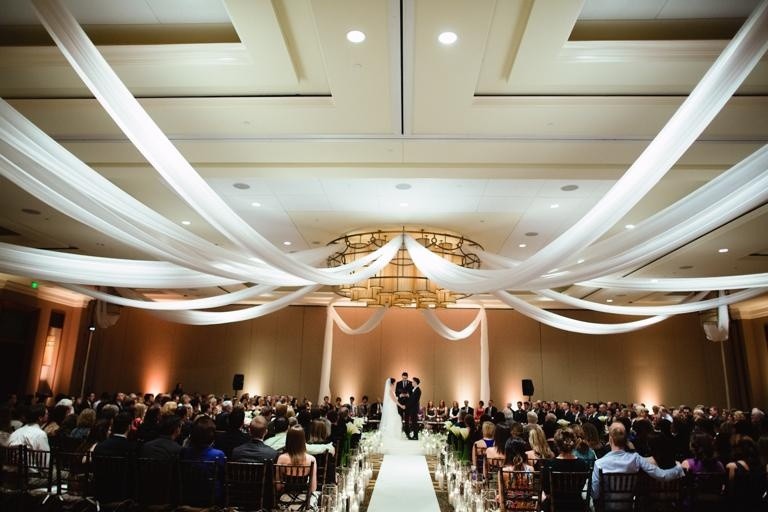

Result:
[522,379,534,396]
[233,374,244,390]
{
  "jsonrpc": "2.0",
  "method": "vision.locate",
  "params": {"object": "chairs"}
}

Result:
[271,458,314,512]
[56,447,93,512]
[475,442,488,474]
[596,464,642,512]
[685,467,729,512]
[527,459,539,467]
[0,443,26,499]
[58,429,87,454]
[24,443,66,498]
[735,471,768,512]
[315,449,328,486]
[222,457,267,512]
[550,467,592,512]
[176,456,221,510]
[134,454,174,510]
[582,458,596,470]
[500,467,544,512]
[642,466,690,512]
[333,440,338,480]
[484,457,504,487]
[91,450,132,512]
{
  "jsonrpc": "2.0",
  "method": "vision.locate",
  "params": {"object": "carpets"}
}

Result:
[364,452,442,512]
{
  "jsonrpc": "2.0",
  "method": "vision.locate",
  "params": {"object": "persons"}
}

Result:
[726,433,767,511]
[436,399,448,431]
[503,399,766,435]
[448,401,460,427]
[92,414,141,490]
[4,392,330,437]
[725,436,763,506]
[358,395,370,416]
[377,377,405,445]
[396,372,414,433]
[496,436,543,512]
[182,416,231,509]
[526,428,556,466]
[339,405,349,426]
[485,400,497,420]
[461,412,478,450]
[346,397,357,414]
[72,418,111,471]
[172,383,184,399]
[460,399,475,417]
[472,420,498,472]
[426,400,437,429]
[674,431,726,512]
[592,421,686,512]
[321,395,333,411]
[333,396,345,413]
[543,426,592,511]
[141,414,187,507]
[567,424,598,464]
[328,408,342,439]
[403,376,422,440]
[485,422,512,469]
[228,414,278,509]
[475,400,484,420]
[6,402,55,482]
[276,423,319,511]
[370,397,382,422]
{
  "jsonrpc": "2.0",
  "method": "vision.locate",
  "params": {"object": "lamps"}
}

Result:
[325,226,484,310]
